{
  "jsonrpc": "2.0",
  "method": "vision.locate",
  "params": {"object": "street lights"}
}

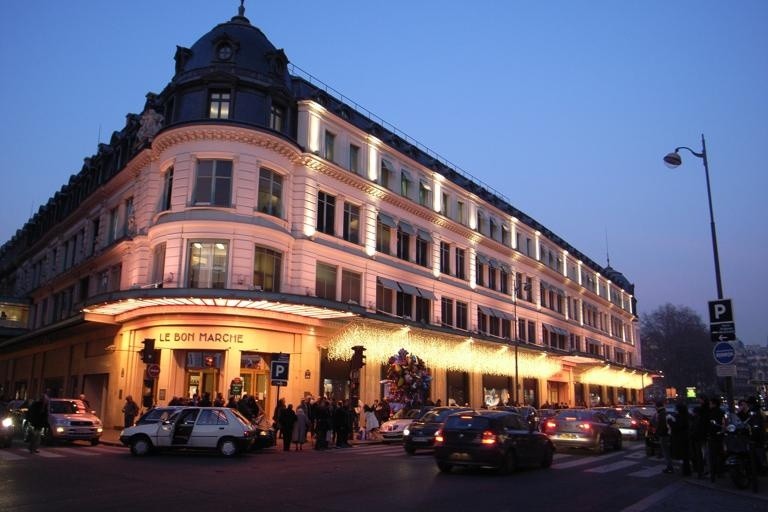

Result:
[642,372,647,404]
[514,279,533,407]
[663,133,736,416]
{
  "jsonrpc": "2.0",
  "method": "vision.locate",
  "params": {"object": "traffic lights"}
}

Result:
[142,338,154,362]
[143,378,152,407]
[353,345,367,369]
[351,376,360,397]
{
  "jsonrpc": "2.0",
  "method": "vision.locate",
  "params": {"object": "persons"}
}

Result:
[595,391,768,484]
[141,386,154,416]
[362,404,381,441]
[79,394,90,408]
[20,395,51,454]
[122,394,139,429]
[168,393,364,453]
[370,399,588,428]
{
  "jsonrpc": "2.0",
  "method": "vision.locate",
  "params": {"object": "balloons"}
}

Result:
[379,347,433,405]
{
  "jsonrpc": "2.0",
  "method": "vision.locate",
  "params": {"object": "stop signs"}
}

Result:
[149,364,160,377]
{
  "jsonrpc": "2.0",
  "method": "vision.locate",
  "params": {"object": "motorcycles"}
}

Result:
[709,411,758,490]
[0,404,22,448]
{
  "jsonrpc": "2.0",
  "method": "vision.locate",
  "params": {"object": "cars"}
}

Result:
[403,406,483,455]
[540,409,622,454]
[119,406,257,457]
[5,399,34,418]
[17,399,104,446]
[432,410,557,476]
[644,409,711,459]
[378,406,434,445]
[135,405,277,452]
[492,400,743,440]
